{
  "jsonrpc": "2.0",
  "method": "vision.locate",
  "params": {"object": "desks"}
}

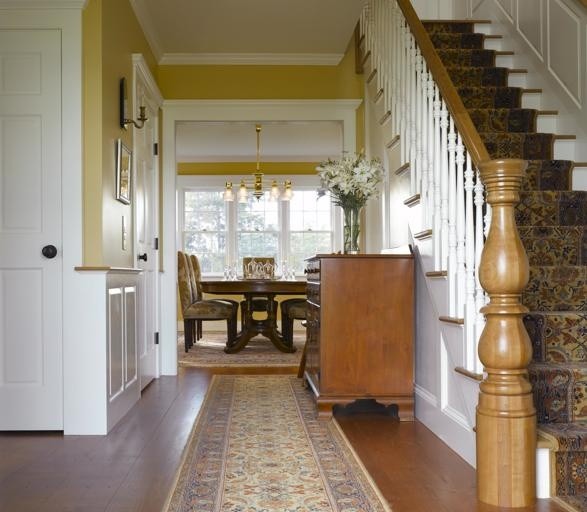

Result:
[200,279,306,354]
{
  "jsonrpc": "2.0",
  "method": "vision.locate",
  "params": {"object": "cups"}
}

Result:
[223,265,237,281]
[279,261,299,280]
[238,264,248,279]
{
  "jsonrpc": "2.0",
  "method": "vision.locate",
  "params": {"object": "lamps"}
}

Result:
[119,78,149,132]
[222,123,294,206]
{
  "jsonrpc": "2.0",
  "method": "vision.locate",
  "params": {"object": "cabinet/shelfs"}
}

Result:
[74,265,142,435]
[300,253,417,423]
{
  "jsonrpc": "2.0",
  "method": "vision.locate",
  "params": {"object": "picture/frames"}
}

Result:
[116,137,132,205]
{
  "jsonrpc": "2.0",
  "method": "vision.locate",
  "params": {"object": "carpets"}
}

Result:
[177,333,307,367]
[161,374,392,512]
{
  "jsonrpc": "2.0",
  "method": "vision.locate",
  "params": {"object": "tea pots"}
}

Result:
[244,258,261,279]
[259,258,275,279]
[252,261,268,278]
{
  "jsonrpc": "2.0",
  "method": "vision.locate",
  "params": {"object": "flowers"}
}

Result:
[315,148,387,242]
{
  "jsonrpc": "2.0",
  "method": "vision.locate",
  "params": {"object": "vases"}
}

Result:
[340,203,363,255]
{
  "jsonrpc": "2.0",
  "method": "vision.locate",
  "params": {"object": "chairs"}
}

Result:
[185,252,237,346]
[239,256,279,328]
[190,254,239,341]
[178,250,233,353]
